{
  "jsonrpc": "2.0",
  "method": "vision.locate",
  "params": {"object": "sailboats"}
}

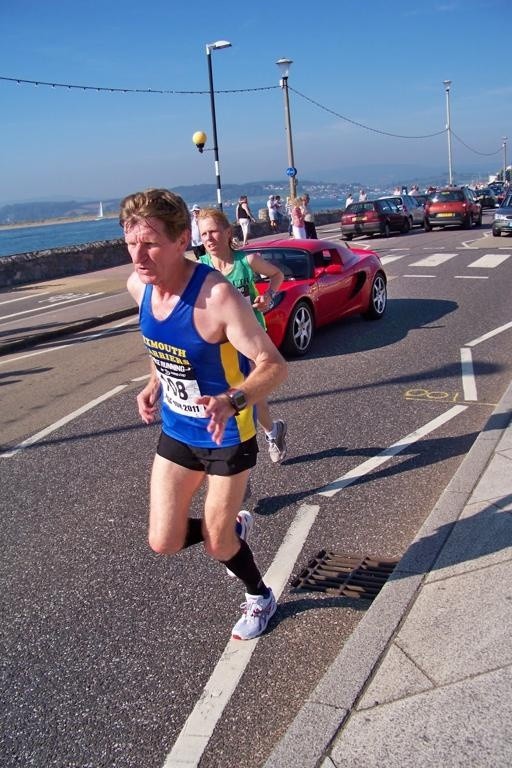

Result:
[94,201,104,221]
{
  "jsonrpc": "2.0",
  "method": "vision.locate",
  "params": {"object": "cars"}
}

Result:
[232,238,388,355]
[341,180,512,238]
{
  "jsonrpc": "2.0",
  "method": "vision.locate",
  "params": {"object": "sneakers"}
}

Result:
[265,419,287,462]
[231,587,278,640]
[227,510,253,577]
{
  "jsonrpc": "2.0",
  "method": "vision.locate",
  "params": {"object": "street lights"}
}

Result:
[274,56,299,205]
[501,136,508,181]
[193,38,232,216]
[441,79,453,185]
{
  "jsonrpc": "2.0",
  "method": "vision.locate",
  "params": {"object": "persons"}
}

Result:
[345,184,436,210]
[235,195,255,245]
[195,207,290,504]
[115,187,290,641]
[267,193,317,239]
[188,204,205,261]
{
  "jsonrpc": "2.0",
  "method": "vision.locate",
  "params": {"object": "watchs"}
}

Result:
[266,287,277,298]
[223,387,249,416]
